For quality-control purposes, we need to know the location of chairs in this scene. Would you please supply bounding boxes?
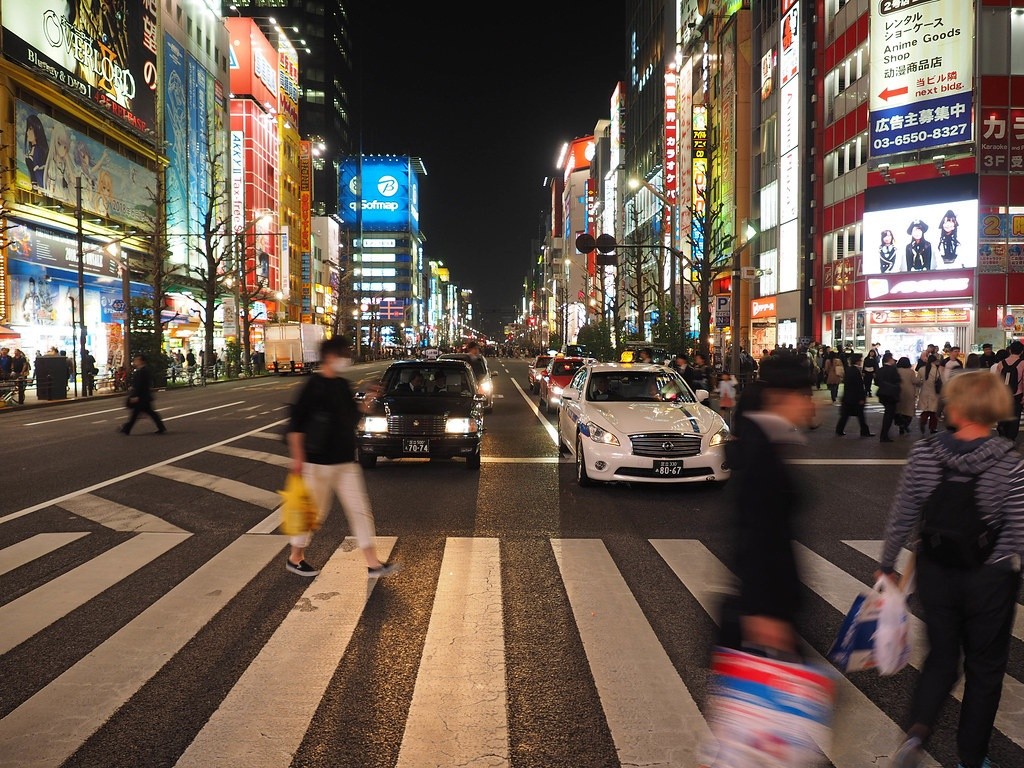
[395,372,411,390]
[446,374,461,385]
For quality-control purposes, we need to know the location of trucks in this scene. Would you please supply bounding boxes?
[263,322,324,376]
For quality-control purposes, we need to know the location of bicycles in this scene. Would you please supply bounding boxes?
[166,367,207,388]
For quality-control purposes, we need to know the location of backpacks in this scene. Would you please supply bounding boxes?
[1001,358,1023,394]
[910,447,1020,622]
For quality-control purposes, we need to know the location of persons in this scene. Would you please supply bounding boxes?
[480,345,528,359]
[118,353,166,436]
[714,371,738,419]
[664,353,715,407]
[86,350,96,396]
[186,349,195,366]
[397,371,426,392]
[284,339,396,578]
[432,371,446,393]
[710,357,813,662]
[388,349,405,360]
[0,348,31,404]
[447,345,461,353]
[416,349,425,358]
[759,340,1024,443]
[463,341,481,379]
[873,370,1024,768]
[33,347,73,390]
[640,348,654,363]
[218,348,226,375]
[162,349,183,369]
[241,348,257,364]
[592,377,613,399]
[877,210,961,273]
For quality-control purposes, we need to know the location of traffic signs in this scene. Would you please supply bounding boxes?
[716,297,730,327]
[868,0,974,162]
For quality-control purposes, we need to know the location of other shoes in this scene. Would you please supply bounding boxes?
[862,432,876,437]
[366,559,400,580]
[115,422,132,436]
[868,392,872,397]
[286,557,318,575]
[155,426,169,433]
[835,430,847,436]
[879,439,894,442]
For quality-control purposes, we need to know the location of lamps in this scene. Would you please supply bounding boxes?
[933,155,950,177]
[878,163,896,184]
[230,5,311,54]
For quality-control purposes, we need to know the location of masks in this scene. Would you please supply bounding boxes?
[331,358,351,373]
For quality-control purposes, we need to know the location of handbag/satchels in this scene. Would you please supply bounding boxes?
[696,646,836,768]
[935,375,943,394]
[835,366,844,377]
[876,578,912,681]
[280,476,321,533]
[829,578,880,675]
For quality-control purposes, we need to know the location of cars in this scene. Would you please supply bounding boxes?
[558,361,731,492]
[528,355,556,395]
[354,360,487,471]
[540,357,597,415]
[430,353,499,415]
